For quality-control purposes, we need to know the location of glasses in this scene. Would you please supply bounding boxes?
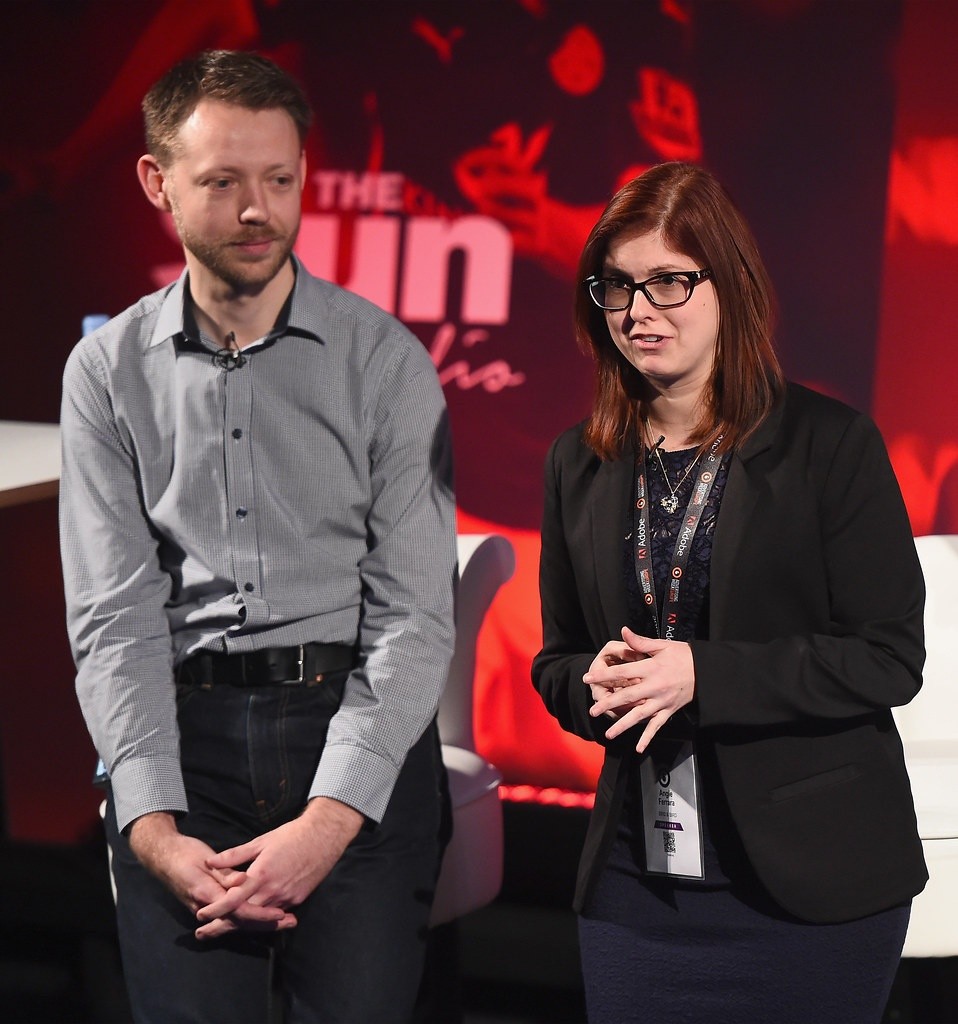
[581,268,714,310]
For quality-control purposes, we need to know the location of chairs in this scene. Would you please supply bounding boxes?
[270,530,520,1024]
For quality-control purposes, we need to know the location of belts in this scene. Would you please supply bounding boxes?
[174,644,359,686]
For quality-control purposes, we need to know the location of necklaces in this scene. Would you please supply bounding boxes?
[646,412,702,512]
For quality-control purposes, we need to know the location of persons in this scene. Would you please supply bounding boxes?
[60,45,461,1024]
[534,164,930,1024]
